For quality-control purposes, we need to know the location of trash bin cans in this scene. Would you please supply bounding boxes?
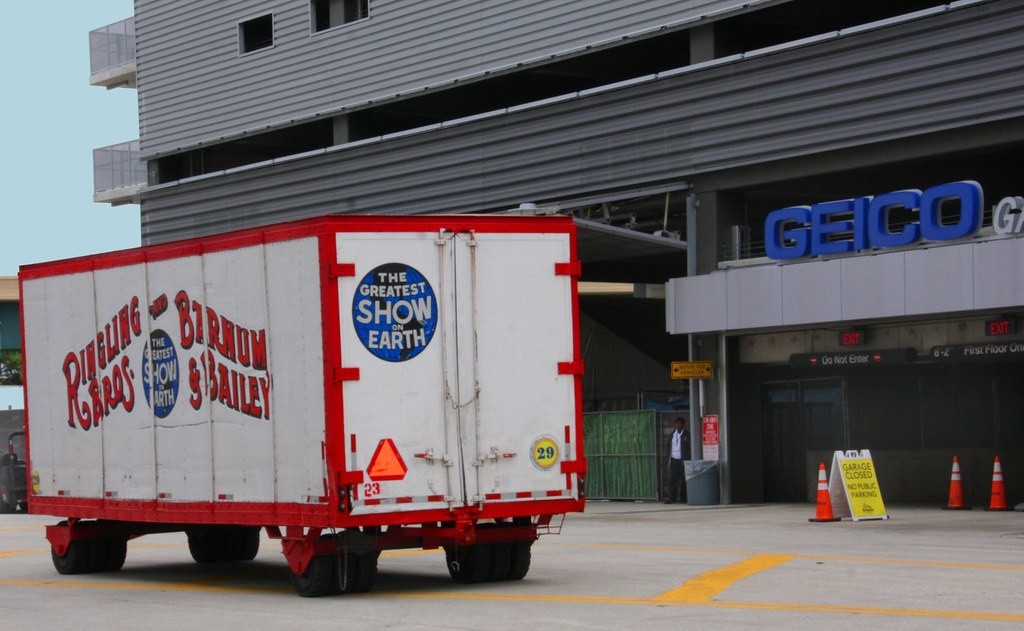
[680,459,720,506]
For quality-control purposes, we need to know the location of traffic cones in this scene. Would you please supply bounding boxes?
[942,456,970,509]
[983,456,1012,511]
[809,462,841,522]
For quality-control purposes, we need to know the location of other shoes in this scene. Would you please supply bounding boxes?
[664,498,673,504]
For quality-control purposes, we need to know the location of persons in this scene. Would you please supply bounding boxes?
[663,417,690,503]
